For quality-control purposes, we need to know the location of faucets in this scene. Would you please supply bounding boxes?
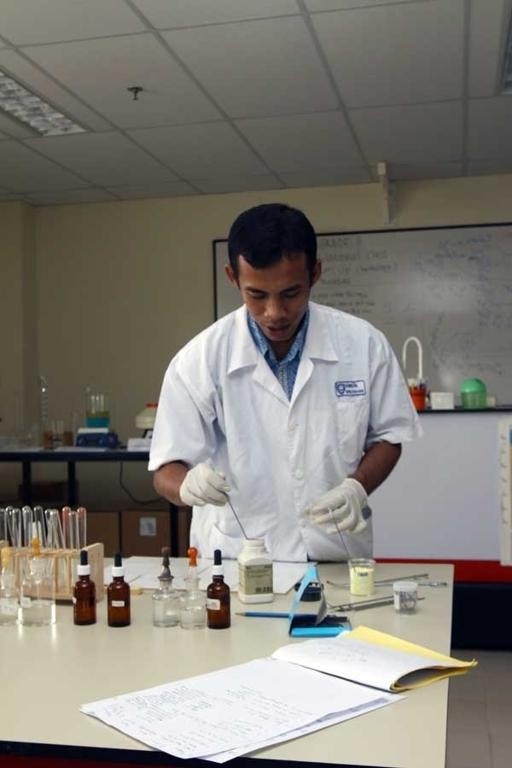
[401,336,423,379]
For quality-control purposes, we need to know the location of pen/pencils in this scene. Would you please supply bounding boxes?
[235,610,291,618]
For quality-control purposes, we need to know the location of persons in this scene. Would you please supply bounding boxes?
[147,200,428,564]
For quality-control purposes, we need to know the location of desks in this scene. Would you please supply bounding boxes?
[1,442,185,557]
[0,554,455,768]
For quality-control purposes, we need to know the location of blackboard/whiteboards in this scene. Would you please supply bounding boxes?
[212,222,510,414]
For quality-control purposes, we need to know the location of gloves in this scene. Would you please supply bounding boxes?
[179,463,231,507]
[310,478,372,535]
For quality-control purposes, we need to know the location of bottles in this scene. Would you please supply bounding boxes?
[2,505,132,628]
[153,544,232,631]
[239,537,273,603]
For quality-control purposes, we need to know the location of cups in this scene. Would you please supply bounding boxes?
[393,580,419,616]
[347,560,375,595]
[406,378,429,411]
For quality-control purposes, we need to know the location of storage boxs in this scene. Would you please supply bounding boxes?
[86,501,191,557]
